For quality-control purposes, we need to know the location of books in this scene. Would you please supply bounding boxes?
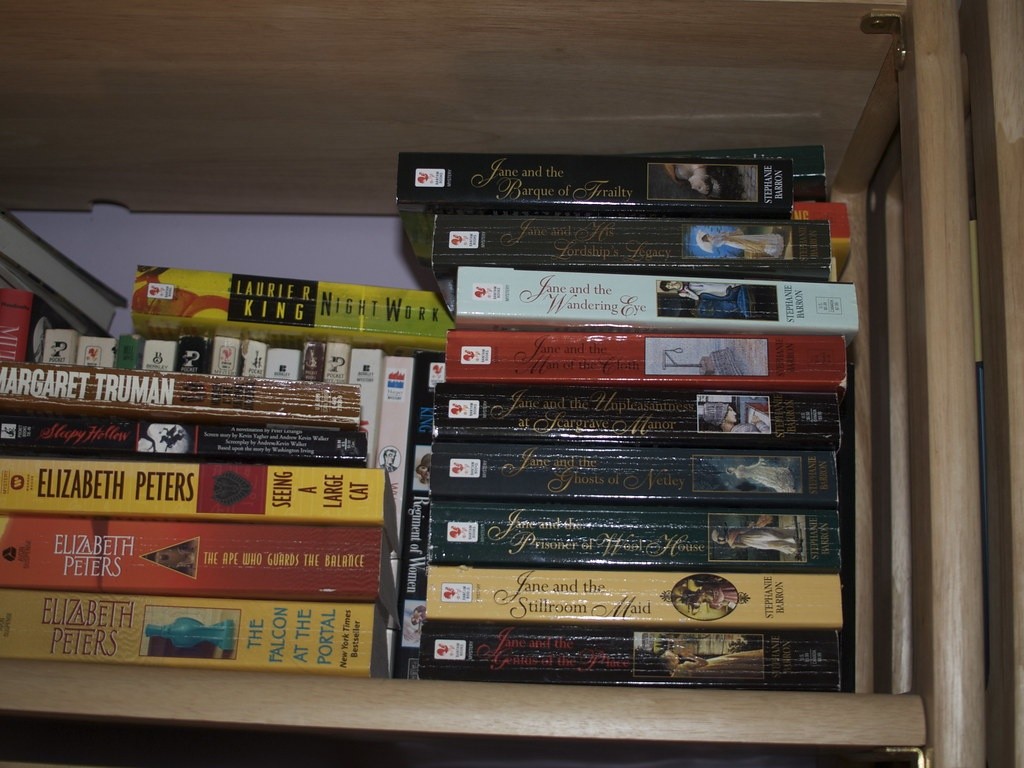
[1,265,455,676]
[395,146,858,692]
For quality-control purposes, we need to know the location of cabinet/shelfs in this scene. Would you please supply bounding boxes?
[0,0,1024,768]
